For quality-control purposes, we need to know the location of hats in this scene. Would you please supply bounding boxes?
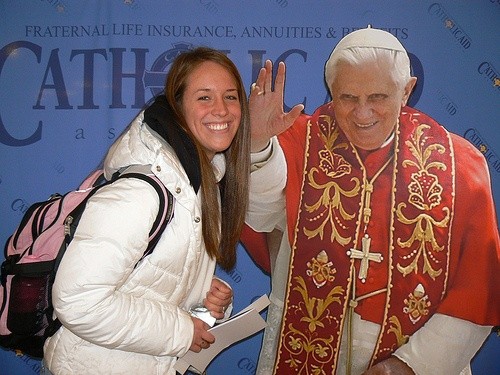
[334,25,407,52]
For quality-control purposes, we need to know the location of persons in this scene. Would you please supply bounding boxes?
[43,46,252,375]
[243,24,500,375]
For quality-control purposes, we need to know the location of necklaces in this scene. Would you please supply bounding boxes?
[346,139,395,280]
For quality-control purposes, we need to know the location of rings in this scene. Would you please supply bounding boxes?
[251,82,265,96]
[222,306,225,313]
[200,340,206,347]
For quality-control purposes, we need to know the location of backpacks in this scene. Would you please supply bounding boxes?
[0,169,176,360]
